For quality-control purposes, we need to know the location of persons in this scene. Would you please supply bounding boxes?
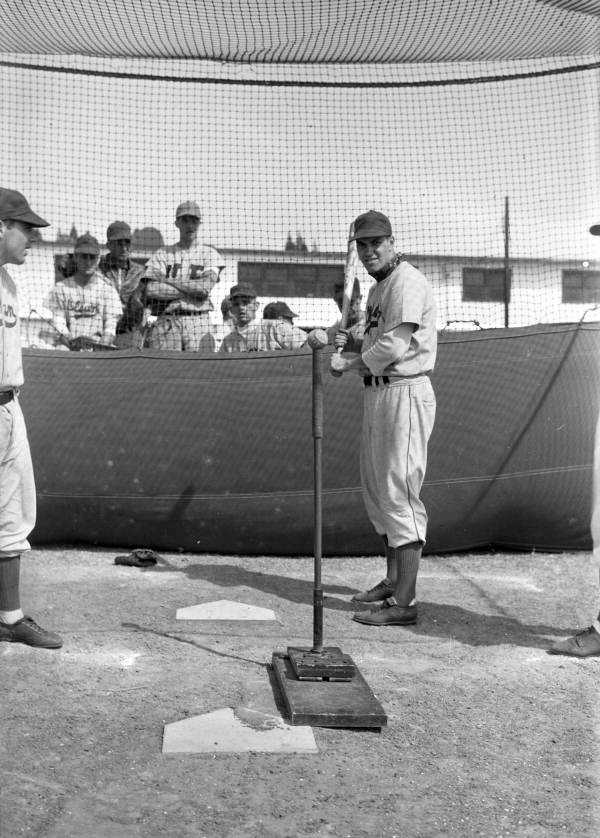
[326,275,367,345]
[329,211,437,625]
[140,200,226,354]
[49,235,123,351]
[263,301,299,325]
[96,221,148,351]
[551,223,599,659]
[219,295,239,339]
[0,186,64,649]
[219,282,310,353]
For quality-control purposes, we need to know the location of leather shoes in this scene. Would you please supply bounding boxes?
[551,625,600,655]
[355,578,395,603]
[354,596,418,626]
[0,616,63,649]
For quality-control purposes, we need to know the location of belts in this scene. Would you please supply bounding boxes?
[164,310,209,316]
[364,376,390,388]
[0,387,20,406]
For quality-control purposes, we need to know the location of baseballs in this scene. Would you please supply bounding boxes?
[308,328,329,349]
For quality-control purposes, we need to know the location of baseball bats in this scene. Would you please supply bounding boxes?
[329,220,360,378]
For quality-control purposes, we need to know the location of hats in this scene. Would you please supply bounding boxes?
[0,186,50,229]
[333,276,361,301]
[107,220,133,243]
[350,209,393,241]
[263,301,299,320]
[230,283,256,301]
[221,295,233,313]
[73,234,101,256]
[176,200,201,220]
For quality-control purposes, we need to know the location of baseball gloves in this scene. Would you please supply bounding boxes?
[114,547,157,567]
